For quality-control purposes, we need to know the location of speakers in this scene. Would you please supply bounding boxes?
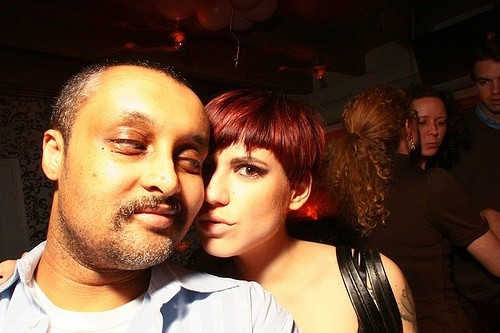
[412,6,500,91]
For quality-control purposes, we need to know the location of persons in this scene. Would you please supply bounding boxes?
[1,91,418,333]
[435,48,500,240]
[327,78,500,333]
[0,59,314,333]
